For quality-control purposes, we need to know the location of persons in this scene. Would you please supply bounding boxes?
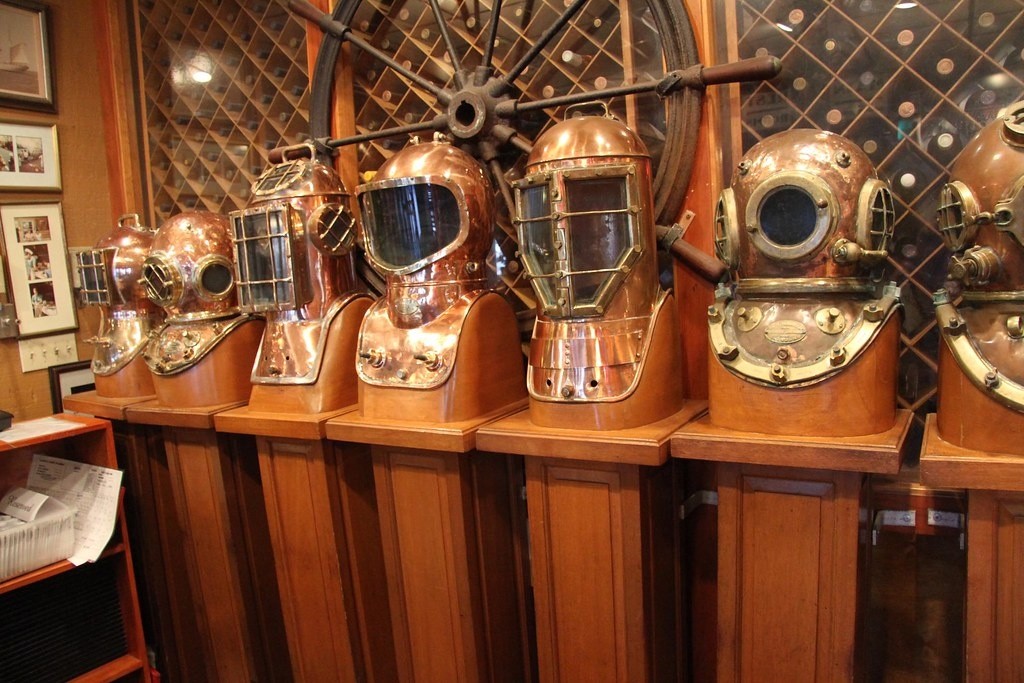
[24,247,39,280]
[25,230,41,241]
[20,150,30,163]
[32,287,46,318]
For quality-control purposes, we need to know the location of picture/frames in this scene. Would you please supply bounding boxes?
[0,1,59,115]
[49,360,96,414]
[0,199,79,340]
[0,117,63,194]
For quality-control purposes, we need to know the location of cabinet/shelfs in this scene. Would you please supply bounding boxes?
[0,411,151,683]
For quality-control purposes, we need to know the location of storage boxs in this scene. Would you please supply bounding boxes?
[0,506,76,583]
